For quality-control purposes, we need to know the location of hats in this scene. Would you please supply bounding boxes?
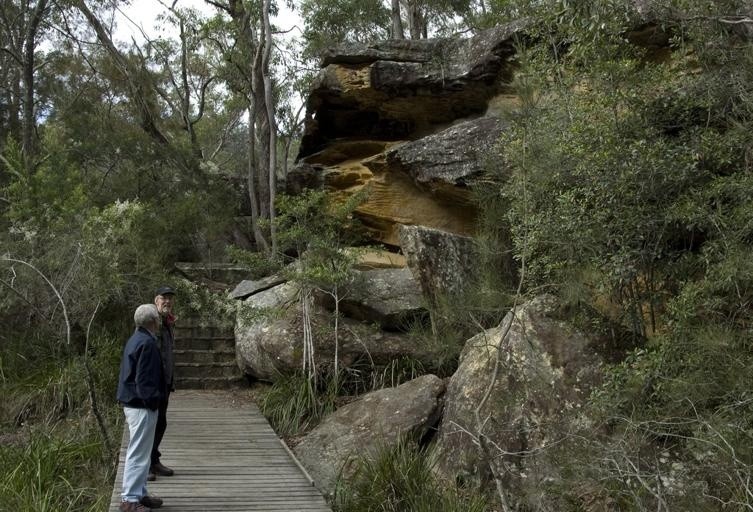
[156,286,175,295]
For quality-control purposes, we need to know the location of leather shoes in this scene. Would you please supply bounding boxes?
[120,496,163,512]
[146,462,175,480]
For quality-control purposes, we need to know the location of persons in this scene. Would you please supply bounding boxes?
[115,286,177,512]
